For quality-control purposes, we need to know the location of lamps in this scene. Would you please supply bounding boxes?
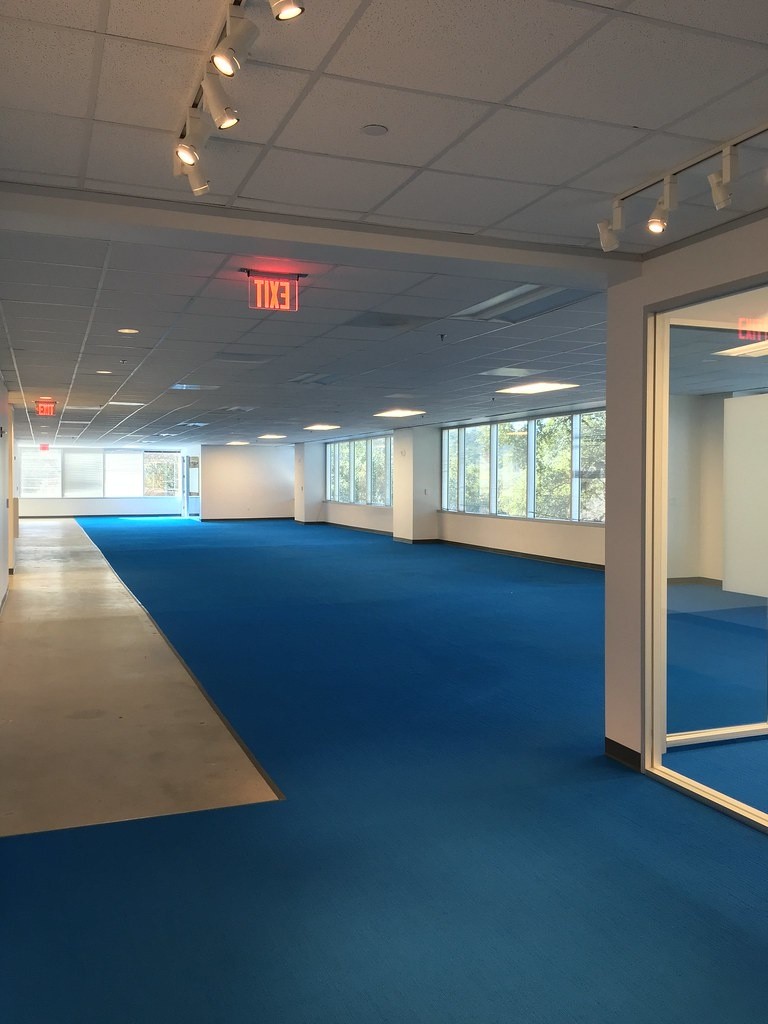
[705,147,741,210]
[210,7,259,78]
[267,0,306,21]
[597,201,626,251]
[197,64,239,130]
[174,109,209,163]
[645,177,679,233]
[179,166,210,196]
